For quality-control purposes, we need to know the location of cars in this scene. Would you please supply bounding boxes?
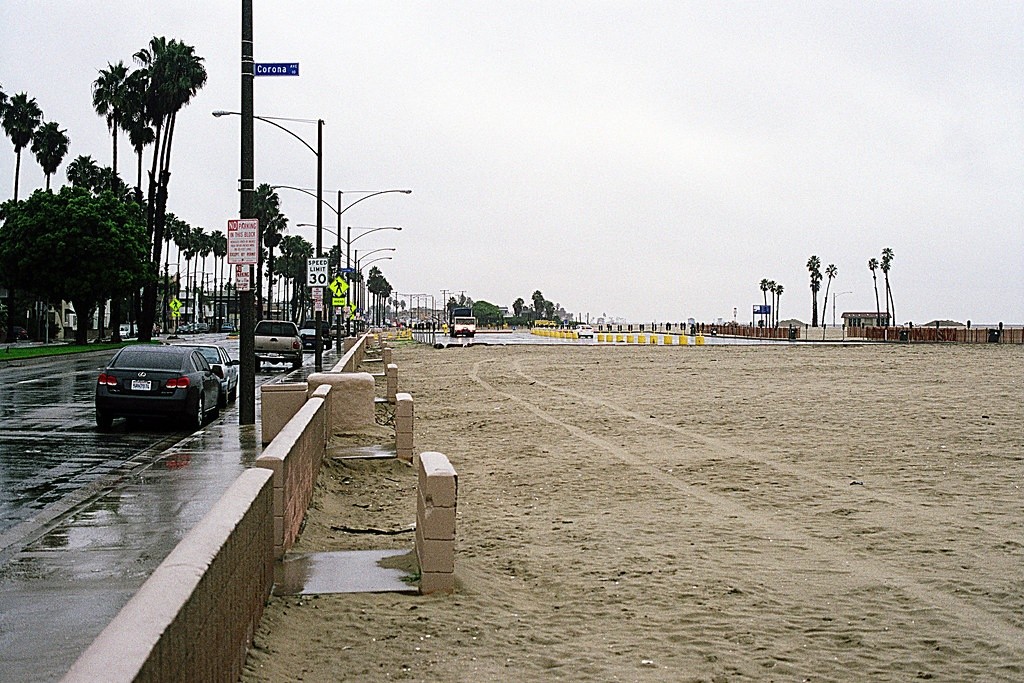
[14,327,28,340]
[576,325,594,339]
[330,320,362,340]
[221,322,234,332]
[170,344,240,408]
[95,344,225,430]
[111,323,210,339]
[298,320,332,350]
[254,320,303,372]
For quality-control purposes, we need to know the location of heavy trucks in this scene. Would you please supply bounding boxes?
[535,320,557,328]
[450,308,478,337]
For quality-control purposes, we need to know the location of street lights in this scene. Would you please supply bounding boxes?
[268,184,412,356]
[212,110,326,369]
[296,223,403,335]
[315,246,397,336]
[327,256,393,331]
